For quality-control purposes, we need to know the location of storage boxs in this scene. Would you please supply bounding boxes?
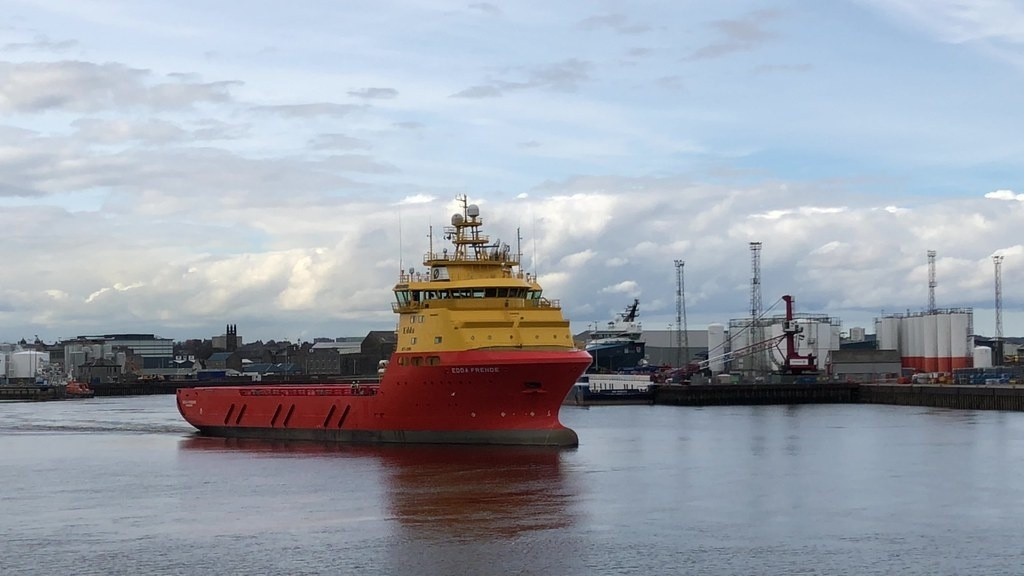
[896,365,1024,386]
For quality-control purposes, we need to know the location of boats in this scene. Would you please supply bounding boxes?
[572,299,652,404]
[177,194,592,447]
